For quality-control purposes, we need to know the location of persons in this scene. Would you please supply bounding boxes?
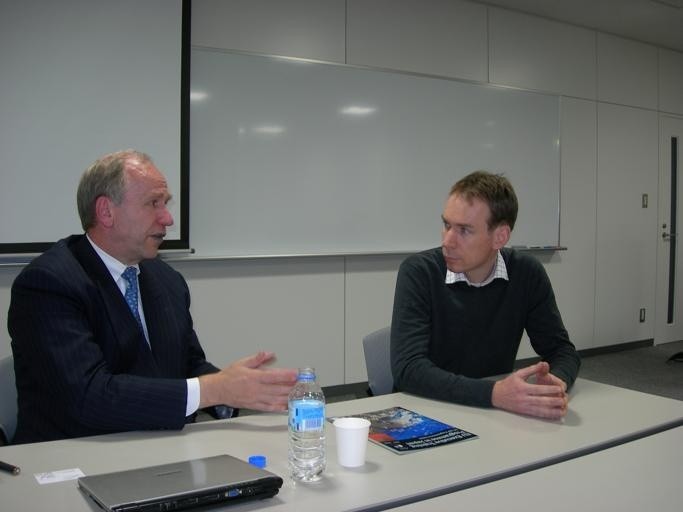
[8,150,299,445]
[391,171,582,420]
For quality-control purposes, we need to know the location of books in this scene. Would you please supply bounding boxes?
[326,406,479,456]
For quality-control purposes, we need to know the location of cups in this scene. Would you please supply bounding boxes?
[332,417,370,466]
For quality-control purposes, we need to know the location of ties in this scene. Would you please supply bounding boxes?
[121,267,142,331]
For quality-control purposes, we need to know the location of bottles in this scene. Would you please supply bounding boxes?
[289,368,327,483]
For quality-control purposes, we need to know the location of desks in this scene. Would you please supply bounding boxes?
[2,370,681,510]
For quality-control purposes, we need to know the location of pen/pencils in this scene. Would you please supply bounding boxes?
[529,247,541,248]
[544,246,557,248]
[511,246,527,248]
[0,461,20,476]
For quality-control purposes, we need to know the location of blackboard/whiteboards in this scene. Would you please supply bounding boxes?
[1,46,567,266]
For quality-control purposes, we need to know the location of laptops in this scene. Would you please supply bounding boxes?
[78,454,283,512]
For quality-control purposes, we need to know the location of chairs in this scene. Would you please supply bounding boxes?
[362,326,395,396]
